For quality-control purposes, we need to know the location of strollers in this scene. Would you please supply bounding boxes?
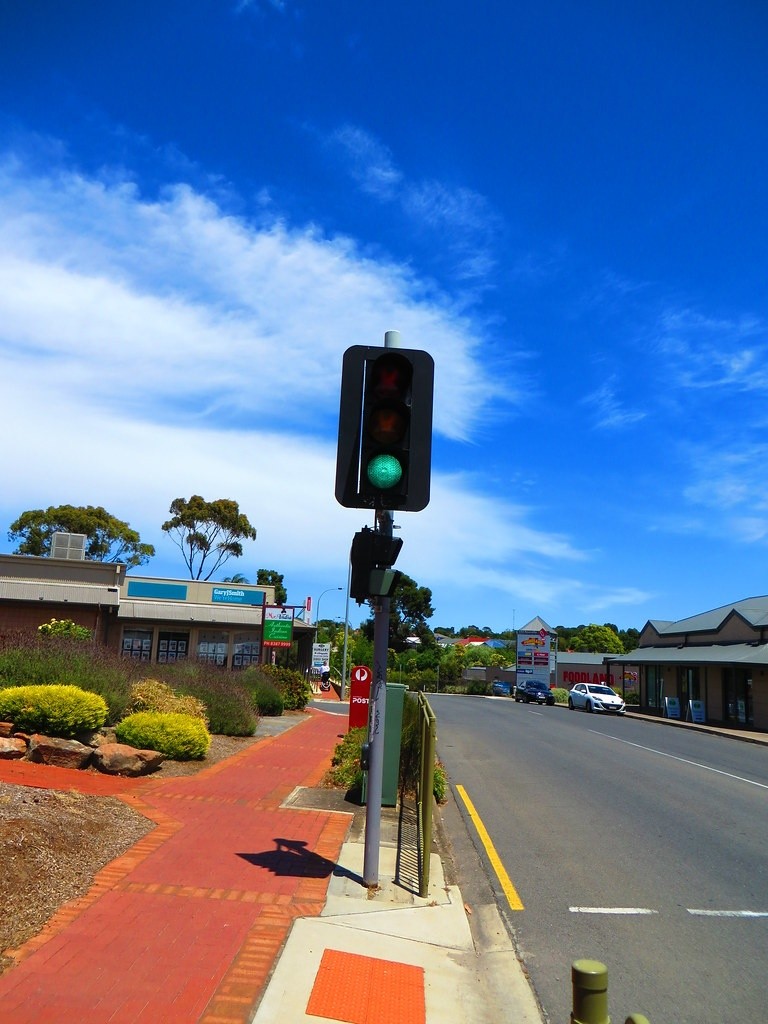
[319,671,331,691]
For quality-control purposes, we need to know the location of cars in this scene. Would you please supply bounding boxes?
[515,679,555,706]
[493,682,509,695]
[568,683,626,715]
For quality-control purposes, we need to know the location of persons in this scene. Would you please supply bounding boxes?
[320,661,331,677]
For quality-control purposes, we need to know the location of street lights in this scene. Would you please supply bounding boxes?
[315,588,343,644]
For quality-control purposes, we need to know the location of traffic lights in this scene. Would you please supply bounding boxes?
[334,345,434,513]
[350,524,404,607]
